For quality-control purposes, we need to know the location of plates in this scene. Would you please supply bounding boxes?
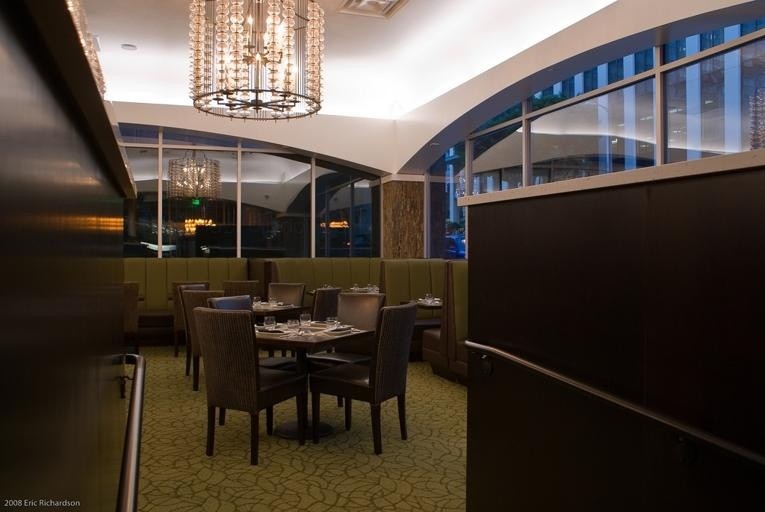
[256,331,290,336]
[325,330,351,335]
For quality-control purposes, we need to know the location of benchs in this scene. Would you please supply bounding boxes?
[123,256,469,387]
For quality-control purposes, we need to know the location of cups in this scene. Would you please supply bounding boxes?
[326,317,337,330]
[253,297,261,305]
[424,293,433,304]
[287,314,312,335]
[264,317,276,330]
[353,287,359,294]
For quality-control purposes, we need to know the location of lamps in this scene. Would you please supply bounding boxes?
[167,142,222,201]
[187,0,325,122]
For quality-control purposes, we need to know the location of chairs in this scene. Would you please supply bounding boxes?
[171,279,415,470]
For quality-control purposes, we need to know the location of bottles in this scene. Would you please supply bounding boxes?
[269,296,277,303]
[368,282,376,293]
